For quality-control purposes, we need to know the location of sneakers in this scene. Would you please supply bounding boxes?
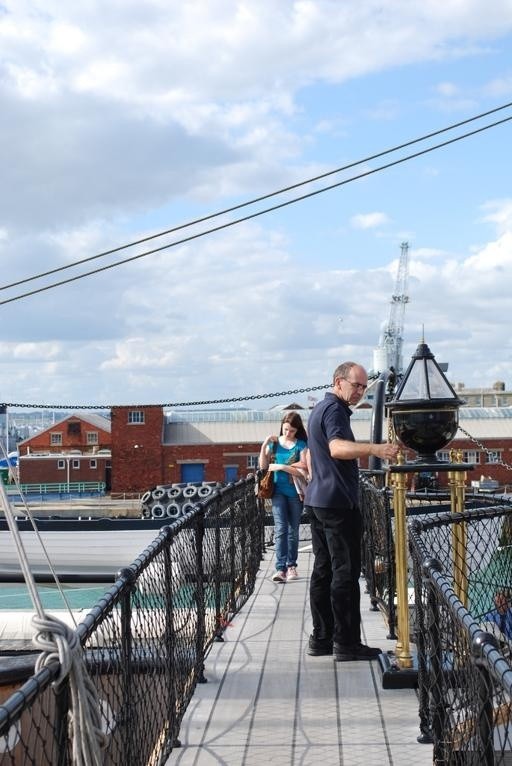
[286,565,298,580]
[332,640,383,662]
[306,633,333,656]
[272,570,287,584]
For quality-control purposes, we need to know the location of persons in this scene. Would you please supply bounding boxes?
[304,361,401,664]
[258,412,309,584]
[479,593,512,643]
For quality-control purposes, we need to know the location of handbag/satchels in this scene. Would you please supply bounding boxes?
[258,435,278,499]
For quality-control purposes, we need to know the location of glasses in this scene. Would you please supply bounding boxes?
[338,377,368,390]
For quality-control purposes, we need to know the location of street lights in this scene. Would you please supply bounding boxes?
[377,321,475,689]
